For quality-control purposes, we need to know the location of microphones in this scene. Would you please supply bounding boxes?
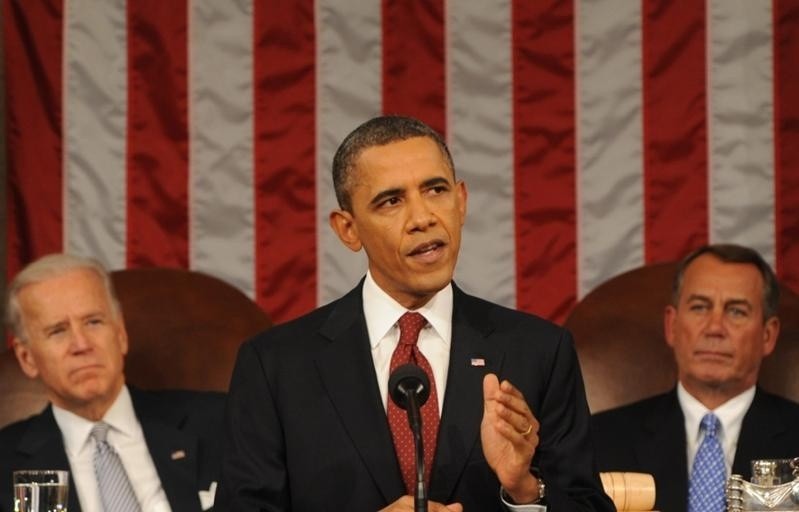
[388,364,431,512]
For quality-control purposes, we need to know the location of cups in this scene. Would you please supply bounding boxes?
[13,470,71,512]
[751,459,799,487]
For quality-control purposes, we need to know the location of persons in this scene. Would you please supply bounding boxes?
[587,244,799,512]
[0,254,230,512]
[223,115,618,512]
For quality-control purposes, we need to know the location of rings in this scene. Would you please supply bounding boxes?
[520,423,533,436]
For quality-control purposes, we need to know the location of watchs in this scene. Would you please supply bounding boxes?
[503,478,546,505]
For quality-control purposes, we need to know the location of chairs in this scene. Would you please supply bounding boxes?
[563,259,798,414]
[0,266,275,429]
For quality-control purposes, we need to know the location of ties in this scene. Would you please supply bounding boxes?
[689,414,727,512]
[386,313,440,497]
[91,423,142,512]
